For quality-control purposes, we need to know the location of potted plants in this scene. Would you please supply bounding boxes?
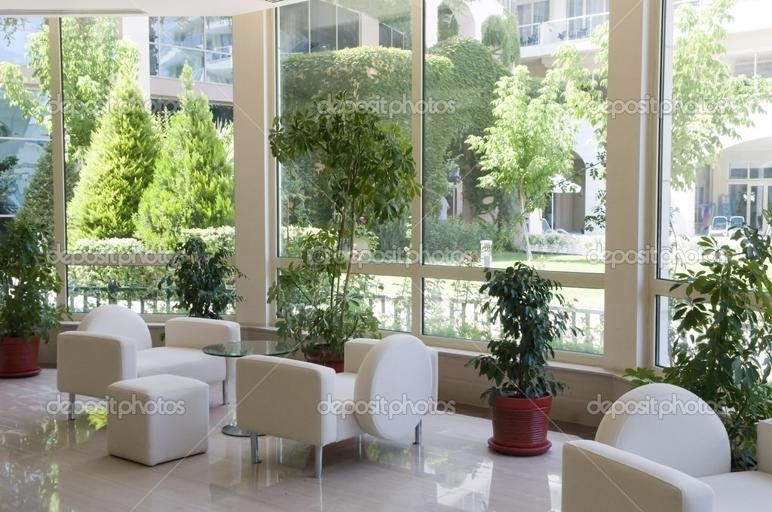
[265,87,424,373]
[464,262,584,454]
[0,210,72,378]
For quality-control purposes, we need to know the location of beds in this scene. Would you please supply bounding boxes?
[203,339,297,437]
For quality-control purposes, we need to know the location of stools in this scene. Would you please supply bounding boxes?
[105,374,210,467]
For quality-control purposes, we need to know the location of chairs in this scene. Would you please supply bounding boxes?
[56,305,240,420]
[561,383,772,512]
[235,335,439,477]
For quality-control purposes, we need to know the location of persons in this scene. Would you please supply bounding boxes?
[437,193,452,221]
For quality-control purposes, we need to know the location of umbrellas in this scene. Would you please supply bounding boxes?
[533,171,582,236]
